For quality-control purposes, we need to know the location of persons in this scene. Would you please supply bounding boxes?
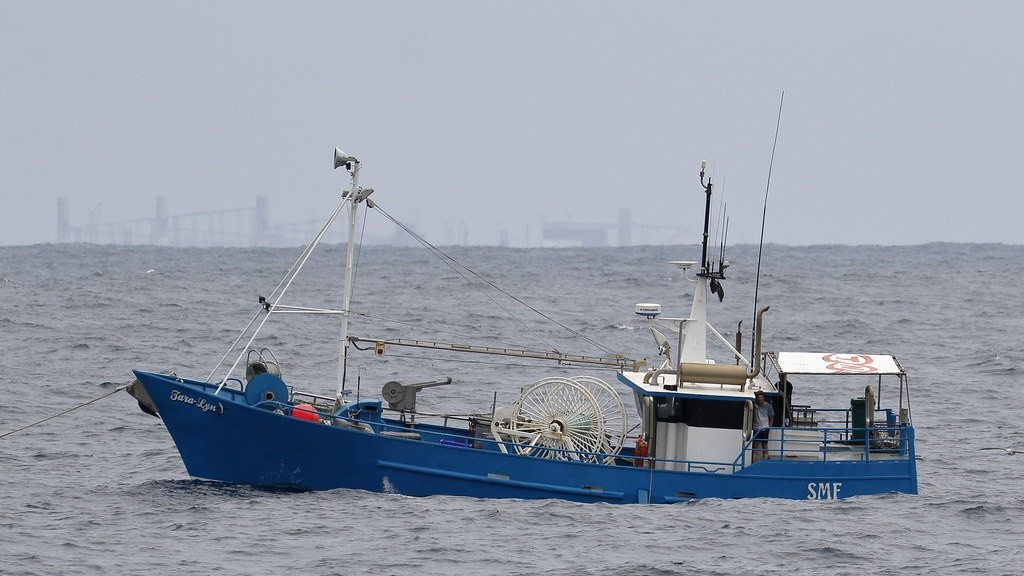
[750,391,775,465]
[772,371,794,427]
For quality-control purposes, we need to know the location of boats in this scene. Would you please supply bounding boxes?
[132,91,920,508]
